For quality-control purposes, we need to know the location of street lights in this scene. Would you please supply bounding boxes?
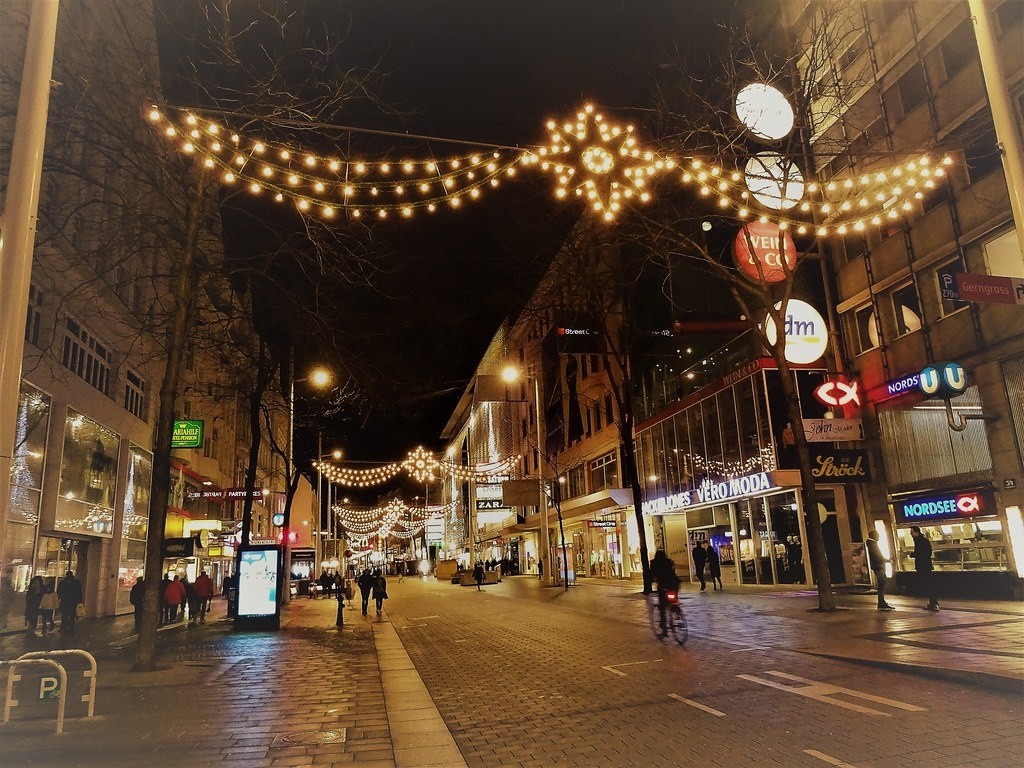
[331,486,349,539]
[415,484,431,575]
[501,365,553,586]
[312,432,343,578]
[447,439,475,569]
[284,366,334,605]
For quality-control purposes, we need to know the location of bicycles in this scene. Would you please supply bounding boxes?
[640,588,689,646]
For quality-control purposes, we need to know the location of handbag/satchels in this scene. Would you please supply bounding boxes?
[76,604,86,620]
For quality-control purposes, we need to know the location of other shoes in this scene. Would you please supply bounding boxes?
[878,605,895,610]
[41,630,46,634]
[700,587,705,590]
[923,604,939,610]
[714,586,716,590]
[49,624,55,631]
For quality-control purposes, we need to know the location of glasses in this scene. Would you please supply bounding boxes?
[911,532,915,533]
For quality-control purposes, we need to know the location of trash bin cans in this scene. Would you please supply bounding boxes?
[227,588,235,618]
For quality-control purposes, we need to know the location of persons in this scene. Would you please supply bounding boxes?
[908,526,941,612]
[485,556,509,576]
[702,539,724,592]
[358,569,388,616]
[320,571,342,596]
[130,577,143,629]
[457,563,463,571]
[160,569,213,626]
[398,572,404,583]
[538,557,543,580]
[865,530,896,611]
[223,570,236,600]
[782,422,796,448]
[23,570,82,634]
[472,559,486,591]
[644,550,683,637]
[692,540,707,591]
